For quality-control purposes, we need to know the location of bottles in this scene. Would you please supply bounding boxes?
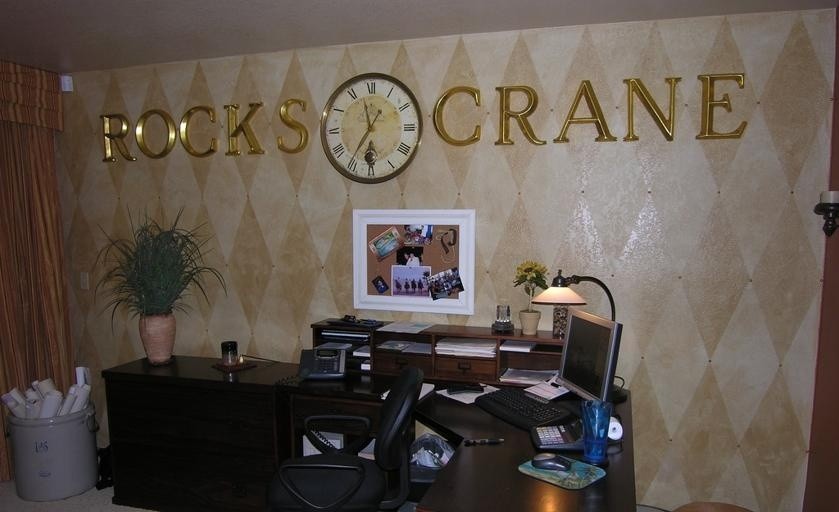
[224,372,238,382]
[239,354,244,364]
[221,340,240,367]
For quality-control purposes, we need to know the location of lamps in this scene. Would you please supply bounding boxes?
[812,190,839,237]
[530,267,628,404]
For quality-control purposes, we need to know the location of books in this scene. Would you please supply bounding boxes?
[352,346,370,356]
[499,340,536,352]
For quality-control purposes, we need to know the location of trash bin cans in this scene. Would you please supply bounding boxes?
[408,433,456,503]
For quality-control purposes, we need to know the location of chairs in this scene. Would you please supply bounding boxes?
[268,364,425,511]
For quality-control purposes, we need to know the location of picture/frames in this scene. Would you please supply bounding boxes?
[353,209,475,316]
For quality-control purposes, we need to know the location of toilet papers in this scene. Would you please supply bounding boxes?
[607,416,623,443]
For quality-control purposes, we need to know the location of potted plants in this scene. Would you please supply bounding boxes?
[87,194,230,368]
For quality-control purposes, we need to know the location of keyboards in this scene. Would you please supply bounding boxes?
[475,388,571,432]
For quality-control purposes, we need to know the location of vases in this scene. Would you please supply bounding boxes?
[518,309,542,336]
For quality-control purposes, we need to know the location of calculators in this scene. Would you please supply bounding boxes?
[531,424,584,449]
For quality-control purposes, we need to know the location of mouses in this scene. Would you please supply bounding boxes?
[532,453,571,472]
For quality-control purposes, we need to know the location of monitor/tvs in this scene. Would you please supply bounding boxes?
[557,308,623,423]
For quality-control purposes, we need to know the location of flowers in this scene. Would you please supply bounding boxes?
[511,260,549,312]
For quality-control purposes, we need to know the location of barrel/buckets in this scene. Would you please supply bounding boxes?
[4,404,100,501]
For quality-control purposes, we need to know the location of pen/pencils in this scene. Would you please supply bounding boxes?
[464,438,505,447]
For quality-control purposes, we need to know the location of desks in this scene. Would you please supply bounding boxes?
[100,352,301,512]
[286,316,637,512]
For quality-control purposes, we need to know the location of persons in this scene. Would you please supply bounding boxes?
[403,253,410,265]
[404,276,410,293]
[418,279,423,295]
[395,277,402,294]
[411,278,417,292]
[406,253,419,266]
[374,278,387,293]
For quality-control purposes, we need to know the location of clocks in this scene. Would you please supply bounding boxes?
[319,72,424,185]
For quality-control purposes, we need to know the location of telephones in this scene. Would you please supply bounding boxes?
[297,348,346,380]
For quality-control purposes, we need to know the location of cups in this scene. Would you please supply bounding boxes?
[579,399,618,465]
